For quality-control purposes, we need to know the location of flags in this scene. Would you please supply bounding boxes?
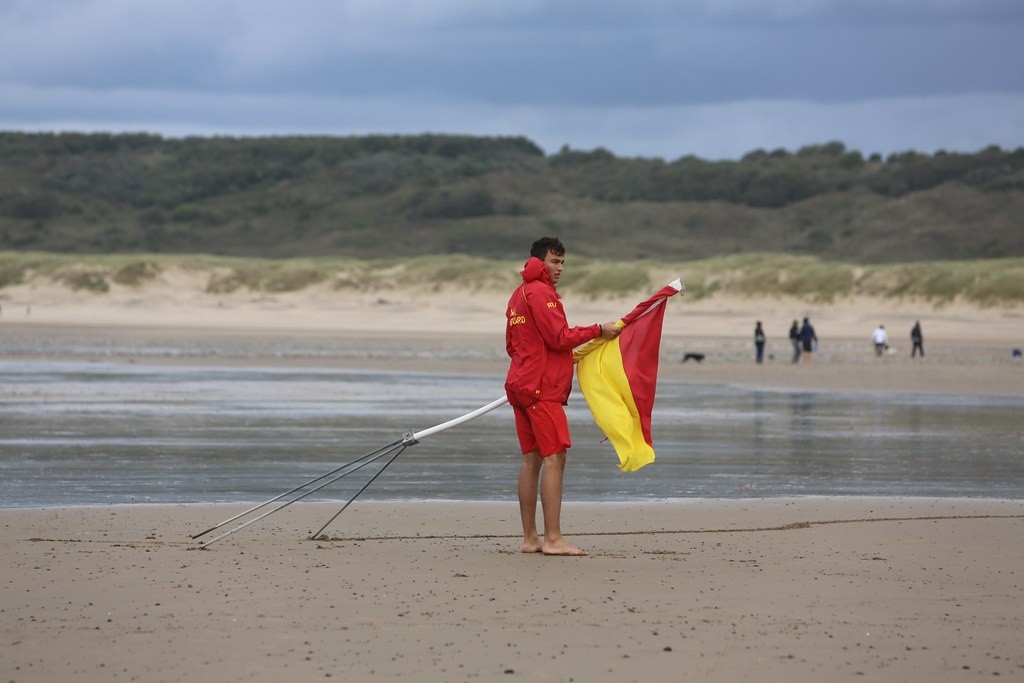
[572,278,682,472]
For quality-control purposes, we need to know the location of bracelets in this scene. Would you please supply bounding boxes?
[599,324,603,337]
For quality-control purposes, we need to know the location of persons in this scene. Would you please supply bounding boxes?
[911,322,924,358]
[504,236,622,555]
[755,322,766,362]
[789,316,818,364]
[872,324,889,357]
